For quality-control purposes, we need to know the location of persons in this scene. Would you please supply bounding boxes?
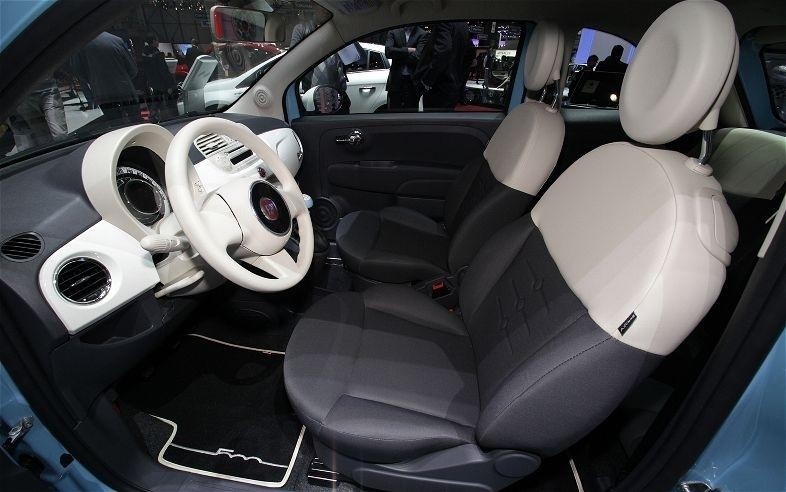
[0,31,203,156]
[569,45,626,98]
[289,9,349,113]
[385,22,496,112]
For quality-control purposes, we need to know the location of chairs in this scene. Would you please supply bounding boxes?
[333,23,567,282]
[705,128,784,272]
[280,2,741,492]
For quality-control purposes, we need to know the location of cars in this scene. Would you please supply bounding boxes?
[174,41,424,116]
[171,36,284,93]
